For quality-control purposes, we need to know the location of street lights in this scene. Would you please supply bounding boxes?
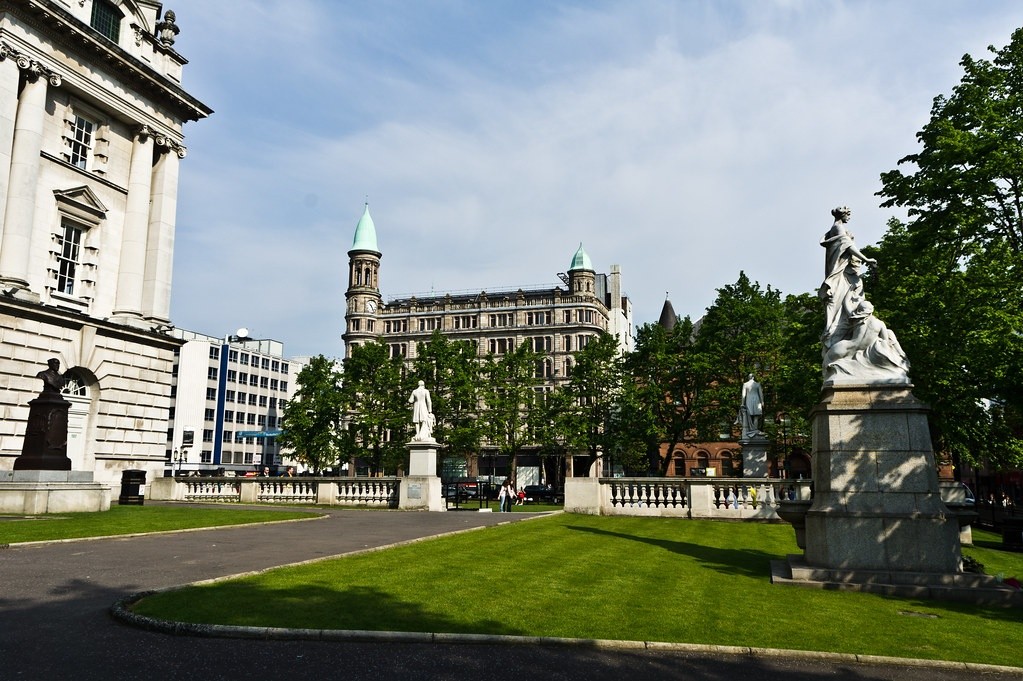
[210,327,252,464]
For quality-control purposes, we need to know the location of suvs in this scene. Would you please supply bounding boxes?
[517,484,555,506]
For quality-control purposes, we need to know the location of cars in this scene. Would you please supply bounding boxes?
[441,483,503,504]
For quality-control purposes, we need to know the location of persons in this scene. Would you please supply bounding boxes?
[498,479,518,513]
[283,466,294,477]
[193,471,200,477]
[259,467,273,477]
[409,380,436,435]
[515,487,526,506]
[36,358,65,393]
[741,374,764,430]
[818,207,910,376]
